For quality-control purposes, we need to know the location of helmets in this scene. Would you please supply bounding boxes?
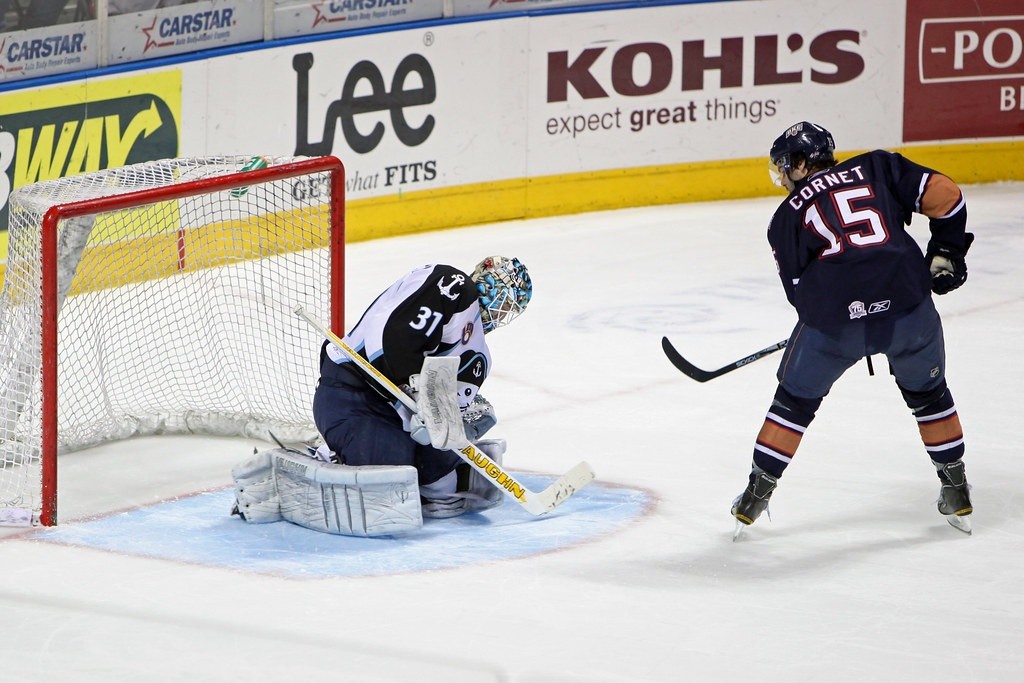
[469,255,532,333]
[770,122,837,170]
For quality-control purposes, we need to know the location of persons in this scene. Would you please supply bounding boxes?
[228,255,531,538]
[729,121,975,538]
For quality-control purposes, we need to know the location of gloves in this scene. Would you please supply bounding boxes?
[924,245,969,294]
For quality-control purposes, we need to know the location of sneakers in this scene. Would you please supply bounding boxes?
[731,472,779,542]
[937,463,974,535]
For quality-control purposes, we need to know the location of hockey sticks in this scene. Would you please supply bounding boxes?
[660,269,955,384]
[291,302,598,519]
